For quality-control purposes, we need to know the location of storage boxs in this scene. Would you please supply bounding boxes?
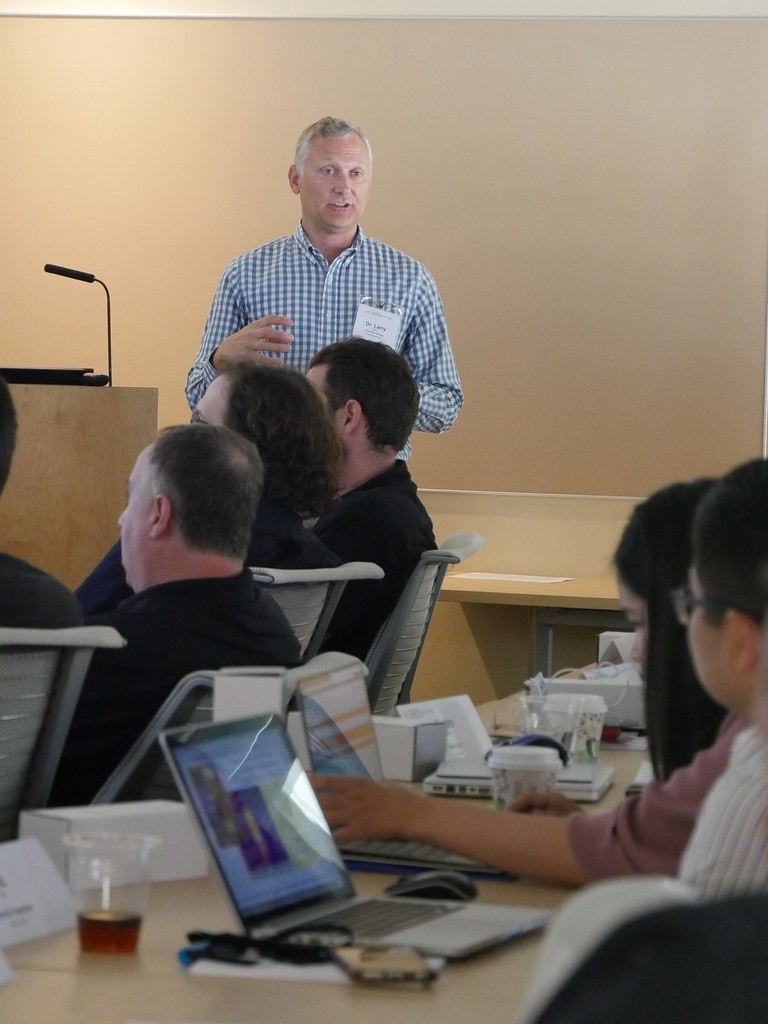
[374,710,454,780]
[16,798,216,890]
[597,631,638,671]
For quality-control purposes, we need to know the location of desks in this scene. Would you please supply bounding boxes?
[0,686,657,1024]
[437,570,635,678]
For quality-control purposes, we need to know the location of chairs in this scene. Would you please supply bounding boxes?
[365,531,488,717]
[0,625,128,842]
[88,651,370,805]
[248,561,385,663]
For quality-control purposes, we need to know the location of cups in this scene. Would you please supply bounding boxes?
[517,695,583,763]
[489,746,562,812]
[64,834,160,953]
[543,694,608,763]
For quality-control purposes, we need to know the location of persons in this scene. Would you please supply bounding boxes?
[184,115,465,461]
[306,458,768,900]
[0,336,438,806]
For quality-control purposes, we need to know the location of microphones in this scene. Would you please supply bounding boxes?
[43,264,112,387]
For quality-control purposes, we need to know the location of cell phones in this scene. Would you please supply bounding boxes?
[357,946,436,983]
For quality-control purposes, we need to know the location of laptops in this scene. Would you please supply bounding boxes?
[159,711,554,958]
[424,760,617,801]
[296,662,520,880]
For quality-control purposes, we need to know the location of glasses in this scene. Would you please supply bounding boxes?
[672,587,714,624]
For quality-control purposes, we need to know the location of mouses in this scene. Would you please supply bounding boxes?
[485,734,569,765]
[385,872,479,902]
